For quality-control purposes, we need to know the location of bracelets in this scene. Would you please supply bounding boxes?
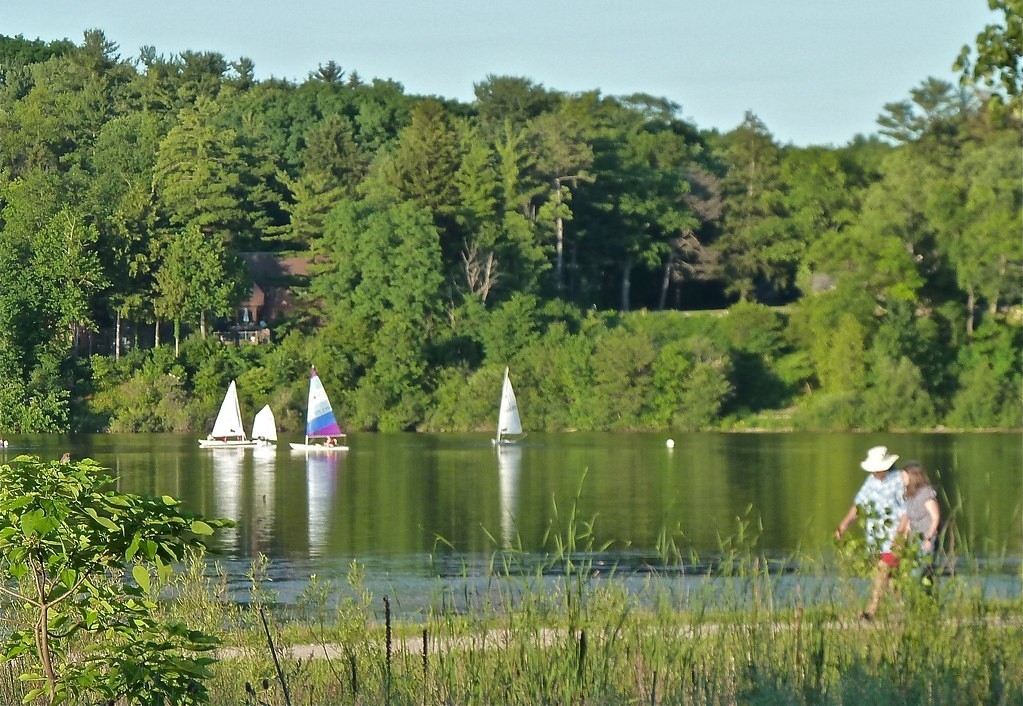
[925,538,930,541]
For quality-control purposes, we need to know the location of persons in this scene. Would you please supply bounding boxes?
[324,436,337,445]
[249,321,254,325]
[221,437,228,441]
[207,432,216,441]
[836,445,901,622]
[891,462,941,597]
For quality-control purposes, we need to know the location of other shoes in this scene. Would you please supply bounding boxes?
[860,611,874,621]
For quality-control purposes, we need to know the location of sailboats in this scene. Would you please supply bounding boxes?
[196,379,256,450]
[249,402,280,452]
[286,364,349,453]
[490,369,530,448]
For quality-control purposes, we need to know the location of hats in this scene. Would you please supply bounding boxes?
[860,446,899,472]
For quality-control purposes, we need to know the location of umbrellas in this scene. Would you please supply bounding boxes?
[243,307,249,322]
